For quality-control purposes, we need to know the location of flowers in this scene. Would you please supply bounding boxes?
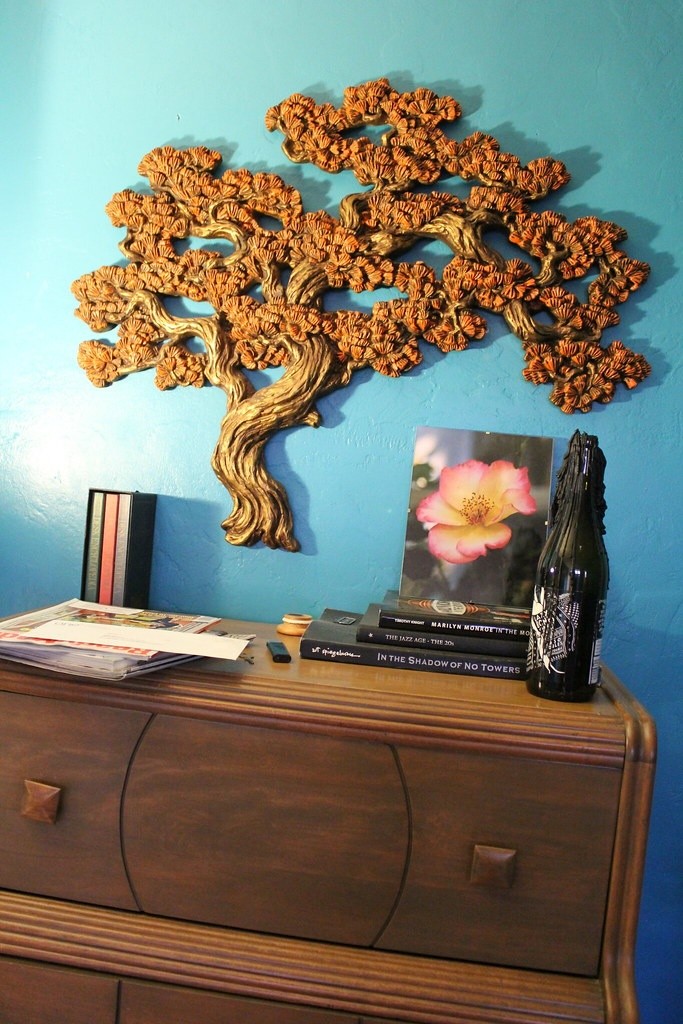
[416,459,538,566]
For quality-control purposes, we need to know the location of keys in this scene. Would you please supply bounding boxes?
[240,652,255,664]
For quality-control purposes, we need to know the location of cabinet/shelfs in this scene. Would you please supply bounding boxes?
[0,607,656,1024]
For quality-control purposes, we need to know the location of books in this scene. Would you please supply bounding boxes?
[0,598,257,681]
[301,589,531,680]
[85,492,130,608]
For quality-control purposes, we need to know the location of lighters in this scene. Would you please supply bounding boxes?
[267,640,291,663]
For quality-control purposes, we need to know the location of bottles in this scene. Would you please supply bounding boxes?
[526,435,609,704]
